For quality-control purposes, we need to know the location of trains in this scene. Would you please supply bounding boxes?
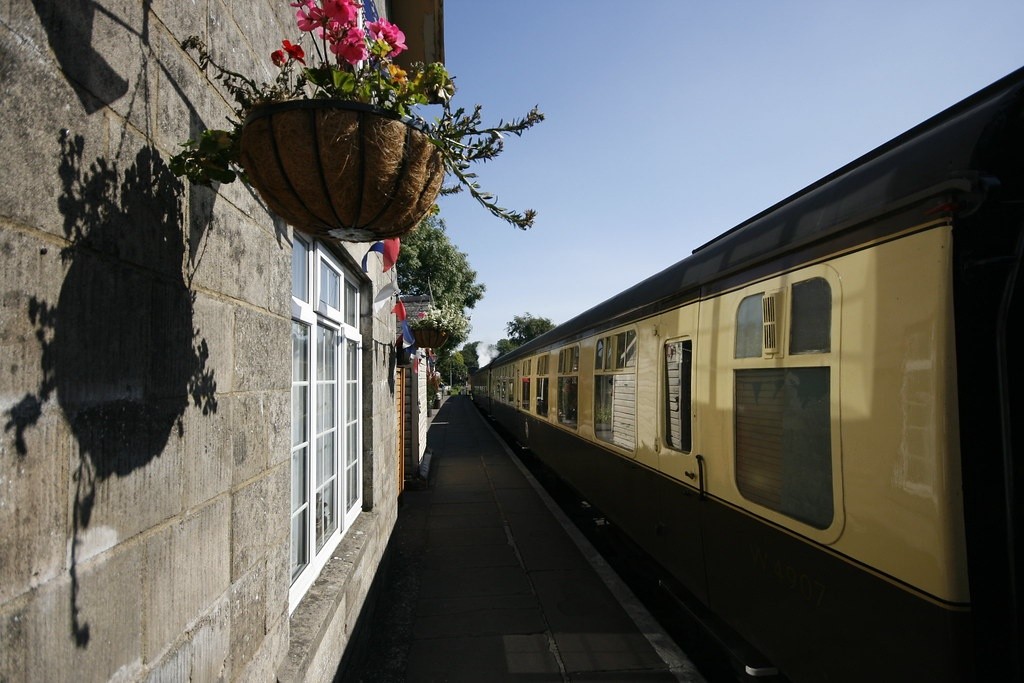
[468,62,1023,683]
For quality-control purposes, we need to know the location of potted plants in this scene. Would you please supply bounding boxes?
[426,369,442,418]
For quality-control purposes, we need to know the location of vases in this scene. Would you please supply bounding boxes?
[412,327,448,348]
[239,98,445,243]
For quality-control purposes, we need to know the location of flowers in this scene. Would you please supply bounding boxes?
[169,0,546,230]
[408,304,459,330]
